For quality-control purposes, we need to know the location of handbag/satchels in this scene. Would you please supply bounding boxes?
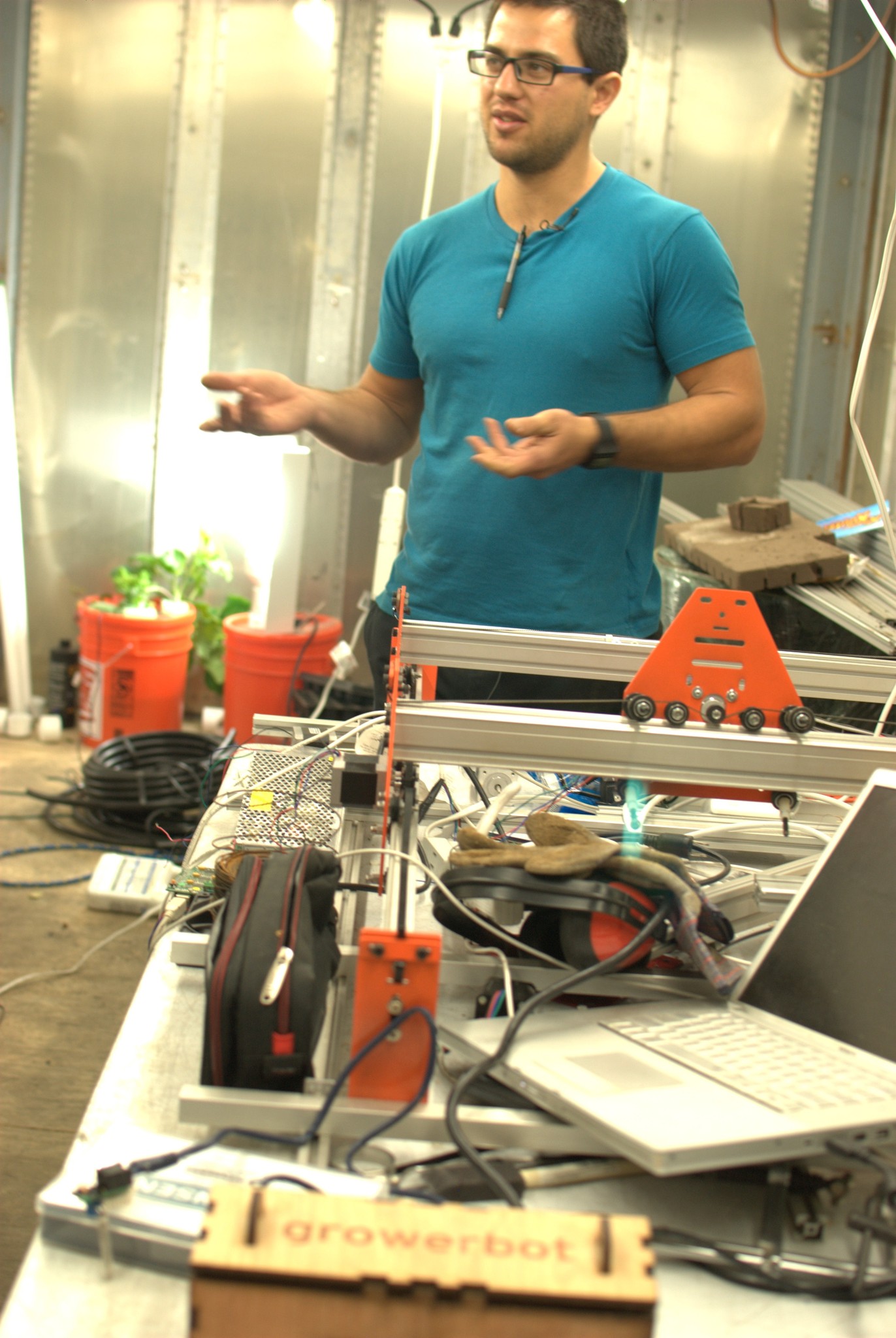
[199,845,342,1092]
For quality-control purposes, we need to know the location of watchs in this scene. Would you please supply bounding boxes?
[577,412,620,470]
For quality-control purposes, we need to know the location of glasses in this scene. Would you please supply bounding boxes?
[467,50,597,85]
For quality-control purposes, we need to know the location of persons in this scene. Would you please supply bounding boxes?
[197,0,766,714]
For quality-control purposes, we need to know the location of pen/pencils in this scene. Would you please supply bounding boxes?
[495,223,528,322]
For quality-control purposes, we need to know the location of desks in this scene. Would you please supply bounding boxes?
[0,733,896,1337]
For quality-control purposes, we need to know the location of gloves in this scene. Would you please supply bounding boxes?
[450,813,746,987]
